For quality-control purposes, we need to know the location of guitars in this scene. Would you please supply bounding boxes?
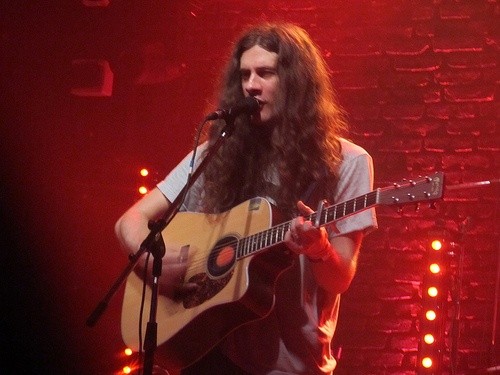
[120,157,447,370]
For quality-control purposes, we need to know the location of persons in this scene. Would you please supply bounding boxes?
[114,23,380,375]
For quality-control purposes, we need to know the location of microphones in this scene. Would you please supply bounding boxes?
[206,96,260,121]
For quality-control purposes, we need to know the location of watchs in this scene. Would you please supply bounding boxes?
[304,248,334,265]
